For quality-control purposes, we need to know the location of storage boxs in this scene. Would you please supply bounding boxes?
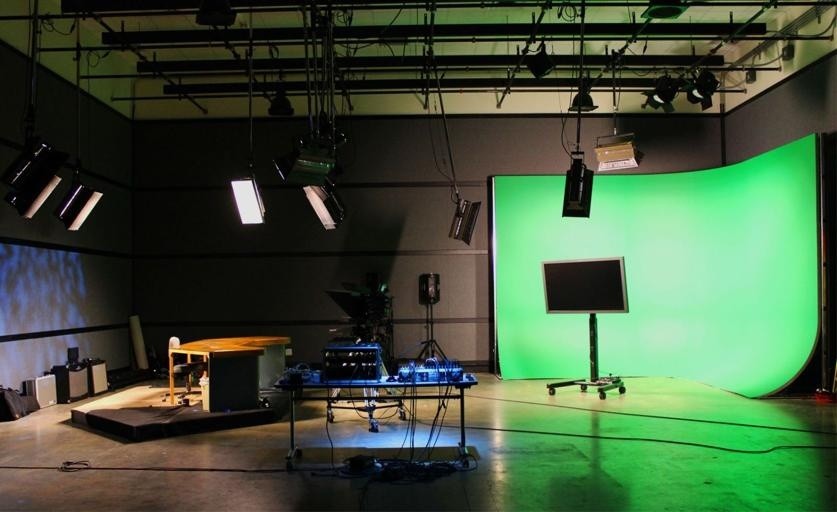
[88,359,111,394]
[54,365,90,404]
[25,375,58,407]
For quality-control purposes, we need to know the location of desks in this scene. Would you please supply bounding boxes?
[282,366,478,470]
[167,333,291,413]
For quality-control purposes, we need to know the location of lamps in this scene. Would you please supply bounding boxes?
[593,38,645,170]
[303,2,348,232]
[5,0,63,221]
[228,1,269,226]
[415,271,451,367]
[561,0,595,218]
[53,1,105,234]
[641,0,777,111]
[424,0,483,245]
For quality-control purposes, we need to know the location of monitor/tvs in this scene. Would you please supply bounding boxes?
[541,256,629,314]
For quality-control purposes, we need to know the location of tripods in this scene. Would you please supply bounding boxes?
[416,304,448,362]
[327,341,406,432]
